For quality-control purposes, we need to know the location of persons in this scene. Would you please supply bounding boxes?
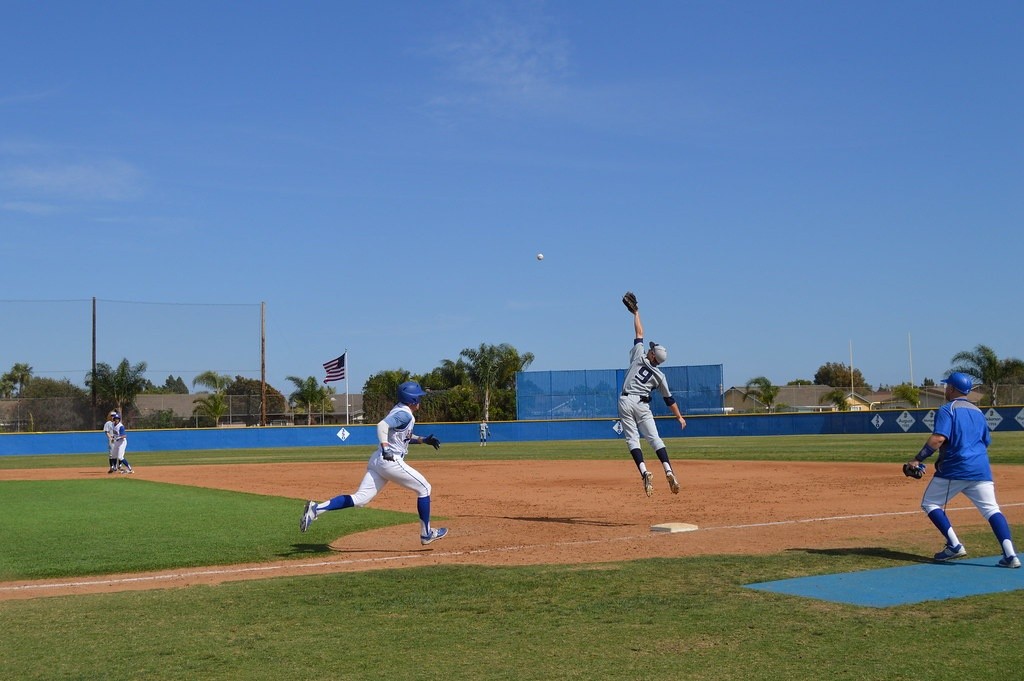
[300,380,449,545]
[618,291,686,497]
[104,412,135,474]
[906,372,1022,567]
[479,419,491,446]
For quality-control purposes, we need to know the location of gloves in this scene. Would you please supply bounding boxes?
[422,434,440,450]
[382,446,395,462]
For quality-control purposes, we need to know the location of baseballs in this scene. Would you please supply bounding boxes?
[537,254,543,260]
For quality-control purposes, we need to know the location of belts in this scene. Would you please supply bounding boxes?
[622,393,642,398]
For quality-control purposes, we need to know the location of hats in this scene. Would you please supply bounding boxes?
[940,371,973,395]
[114,414,121,419]
[111,412,116,416]
[649,341,667,364]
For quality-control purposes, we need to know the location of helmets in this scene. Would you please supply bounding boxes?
[397,382,428,406]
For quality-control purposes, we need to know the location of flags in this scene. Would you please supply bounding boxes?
[323,353,345,384]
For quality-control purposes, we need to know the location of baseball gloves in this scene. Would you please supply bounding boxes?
[902,464,927,479]
[621,291,639,315]
[488,432,491,436]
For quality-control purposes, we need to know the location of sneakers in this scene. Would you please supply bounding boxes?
[299,500,318,533]
[999,554,1021,568]
[420,528,448,545]
[934,544,967,561]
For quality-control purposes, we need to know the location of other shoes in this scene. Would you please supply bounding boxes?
[666,470,680,494]
[642,471,654,497]
